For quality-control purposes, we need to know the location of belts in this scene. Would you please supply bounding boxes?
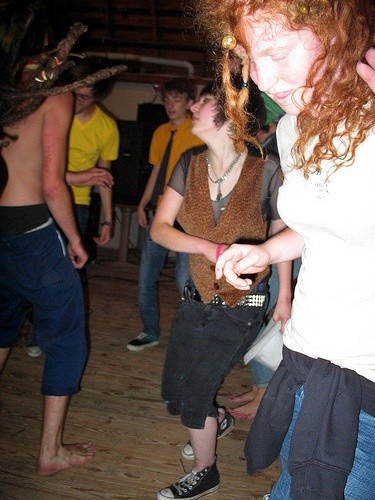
[183,281,270,308]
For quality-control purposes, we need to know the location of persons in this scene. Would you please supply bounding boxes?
[190,0,375,500]
[0,56,119,476]
[127,72,293,499]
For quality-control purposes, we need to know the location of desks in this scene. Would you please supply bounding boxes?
[110,203,150,267]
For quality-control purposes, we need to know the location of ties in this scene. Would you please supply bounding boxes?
[150,129,177,211]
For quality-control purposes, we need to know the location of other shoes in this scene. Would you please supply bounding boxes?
[263,494,270,500]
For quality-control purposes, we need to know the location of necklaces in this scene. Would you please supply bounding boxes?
[205,151,242,200]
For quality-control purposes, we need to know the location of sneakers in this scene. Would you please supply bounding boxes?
[182,408,235,460]
[25,323,43,358]
[127,331,159,351]
[157,456,220,500]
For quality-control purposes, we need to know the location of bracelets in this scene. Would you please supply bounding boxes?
[215,242,223,261]
[101,221,113,227]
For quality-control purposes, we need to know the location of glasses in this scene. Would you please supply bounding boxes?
[74,94,94,100]
